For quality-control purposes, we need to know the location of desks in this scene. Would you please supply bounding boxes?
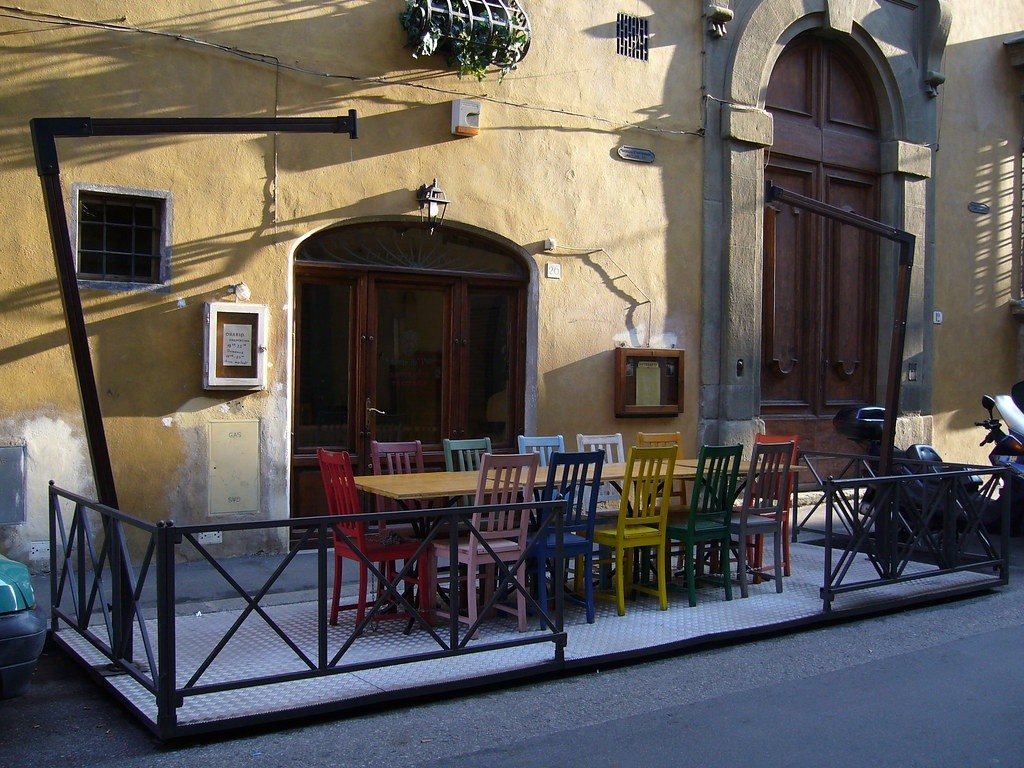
[331,458,810,634]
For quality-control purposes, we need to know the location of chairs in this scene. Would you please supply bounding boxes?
[317,432,798,639]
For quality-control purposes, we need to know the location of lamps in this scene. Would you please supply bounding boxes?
[416,177,451,235]
[227,281,251,300]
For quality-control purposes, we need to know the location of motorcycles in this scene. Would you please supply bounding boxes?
[833,395,1024,552]
